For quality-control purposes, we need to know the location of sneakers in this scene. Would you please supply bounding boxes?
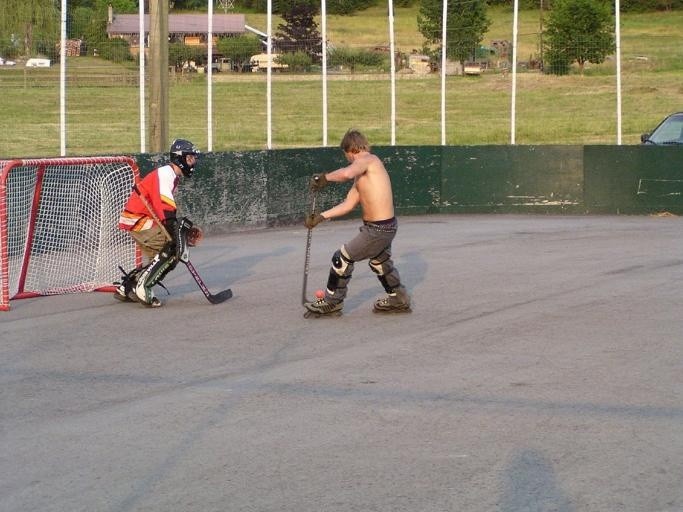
[374,296,409,312]
[304,296,344,314]
[113,284,141,303]
[126,285,162,308]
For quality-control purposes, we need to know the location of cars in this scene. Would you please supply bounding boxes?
[464,62,481,75]
[1,58,15,65]
[641,111,683,145]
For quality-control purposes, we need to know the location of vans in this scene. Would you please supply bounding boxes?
[26,58,51,68]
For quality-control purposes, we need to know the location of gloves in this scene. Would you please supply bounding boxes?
[303,213,324,229]
[308,173,328,192]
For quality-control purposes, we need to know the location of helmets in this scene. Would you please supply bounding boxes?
[169,138,200,179]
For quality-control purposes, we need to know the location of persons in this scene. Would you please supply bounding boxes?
[110,136,205,310]
[298,128,411,314]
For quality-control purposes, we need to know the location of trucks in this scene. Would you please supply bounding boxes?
[205,57,258,74]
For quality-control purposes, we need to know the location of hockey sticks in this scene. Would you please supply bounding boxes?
[131,184,232,303]
[303,177,320,305]
